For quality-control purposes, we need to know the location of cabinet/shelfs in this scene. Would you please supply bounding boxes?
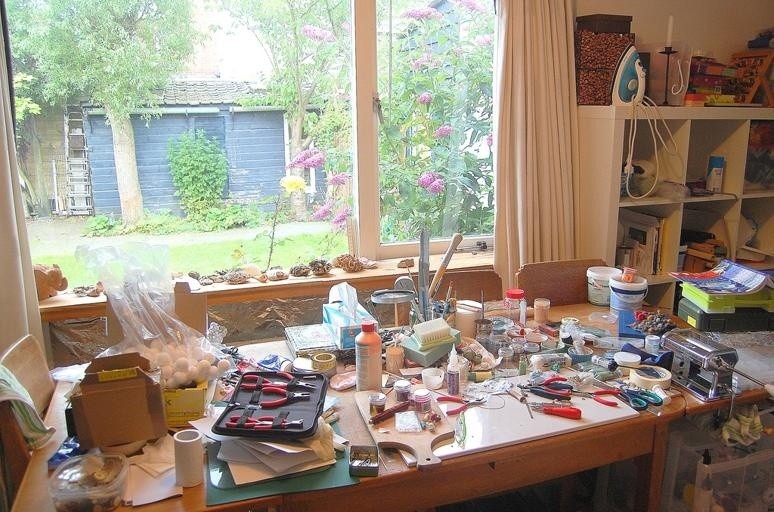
[354,321,383,390]
[502,287,527,328]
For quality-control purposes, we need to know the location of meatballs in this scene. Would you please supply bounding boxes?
[526,402,580,419]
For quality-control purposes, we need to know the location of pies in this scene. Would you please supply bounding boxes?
[480,288,484,324]
[429,233,462,320]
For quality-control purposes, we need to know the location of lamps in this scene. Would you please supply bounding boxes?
[515,259,607,307]
[0,335,56,512]
[408,270,502,307]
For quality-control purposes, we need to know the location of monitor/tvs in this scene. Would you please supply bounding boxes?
[615,209,774,298]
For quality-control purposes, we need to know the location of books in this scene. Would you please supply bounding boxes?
[70,353,169,451]
[576,14,633,34]
[576,70,614,106]
[573,33,636,69]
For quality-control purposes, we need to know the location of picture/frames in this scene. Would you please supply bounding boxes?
[656,316,773,511]
[10,299,683,512]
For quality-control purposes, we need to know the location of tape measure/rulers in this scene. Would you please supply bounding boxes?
[436,395,487,414]
[225,371,316,427]
[524,377,621,406]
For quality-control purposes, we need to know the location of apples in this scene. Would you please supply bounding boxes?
[666,13,674,46]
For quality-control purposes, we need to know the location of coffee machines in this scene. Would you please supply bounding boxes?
[587,377,663,409]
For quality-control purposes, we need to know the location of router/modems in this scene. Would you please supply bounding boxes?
[312,353,336,369]
[540,340,592,362]
[629,364,673,389]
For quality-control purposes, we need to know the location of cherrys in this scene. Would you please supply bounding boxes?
[413,315,457,352]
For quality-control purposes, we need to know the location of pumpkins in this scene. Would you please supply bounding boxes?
[63,103,92,215]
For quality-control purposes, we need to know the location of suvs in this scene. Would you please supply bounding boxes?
[610,276,648,310]
[586,266,622,307]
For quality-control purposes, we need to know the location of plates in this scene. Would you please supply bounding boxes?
[575,106,773,316]
